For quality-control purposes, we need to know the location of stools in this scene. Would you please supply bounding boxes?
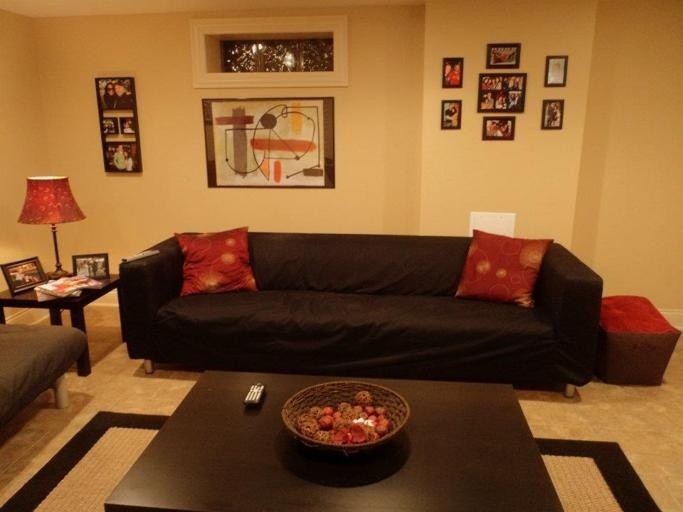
[600,294,682,386]
[1,321,88,450]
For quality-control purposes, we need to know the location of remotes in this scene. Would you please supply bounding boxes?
[122,250,160,264]
[243,382,264,405]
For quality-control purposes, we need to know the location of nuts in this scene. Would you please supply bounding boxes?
[296,390,392,445]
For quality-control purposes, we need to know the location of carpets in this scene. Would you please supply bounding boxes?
[1,409,665,512]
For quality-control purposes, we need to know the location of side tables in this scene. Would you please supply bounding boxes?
[0,271,122,376]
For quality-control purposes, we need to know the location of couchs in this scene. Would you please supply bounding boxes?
[116,231,604,399]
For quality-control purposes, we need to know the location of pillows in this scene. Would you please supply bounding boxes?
[173,225,260,297]
[455,229,554,309]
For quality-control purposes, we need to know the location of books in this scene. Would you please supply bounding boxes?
[34,273,104,302]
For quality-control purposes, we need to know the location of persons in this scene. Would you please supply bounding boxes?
[10,271,38,283]
[104,119,138,171]
[443,47,563,138]
[101,82,132,108]
[114,83,132,109]
[78,260,106,278]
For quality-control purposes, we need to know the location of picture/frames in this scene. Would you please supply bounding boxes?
[201,96,336,189]
[94,77,143,173]
[440,43,569,142]
[0,256,50,297]
[72,251,109,282]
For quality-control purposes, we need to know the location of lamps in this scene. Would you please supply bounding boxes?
[17,175,87,282]
